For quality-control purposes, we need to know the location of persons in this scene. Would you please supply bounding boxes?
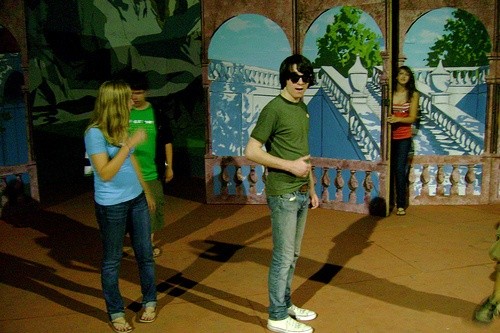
[129,72,173,256]
[245,54,318,333]
[385,66,418,216]
[84,80,158,333]
[474,222,500,322]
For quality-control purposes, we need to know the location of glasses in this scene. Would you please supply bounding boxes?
[132,90,145,95]
[288,72,313,84]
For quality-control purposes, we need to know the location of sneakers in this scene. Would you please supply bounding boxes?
[287,304,316,320]
[267,316,313,333]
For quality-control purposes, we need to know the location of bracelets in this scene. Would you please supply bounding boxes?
[165,162,171,169]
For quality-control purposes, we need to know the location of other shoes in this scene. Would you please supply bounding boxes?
[475,297,498,323]
[152,241,162,256]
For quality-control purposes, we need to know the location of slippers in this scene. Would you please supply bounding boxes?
[138,306,157,323]
[109,317,132,333]
[396,209,406,215]
